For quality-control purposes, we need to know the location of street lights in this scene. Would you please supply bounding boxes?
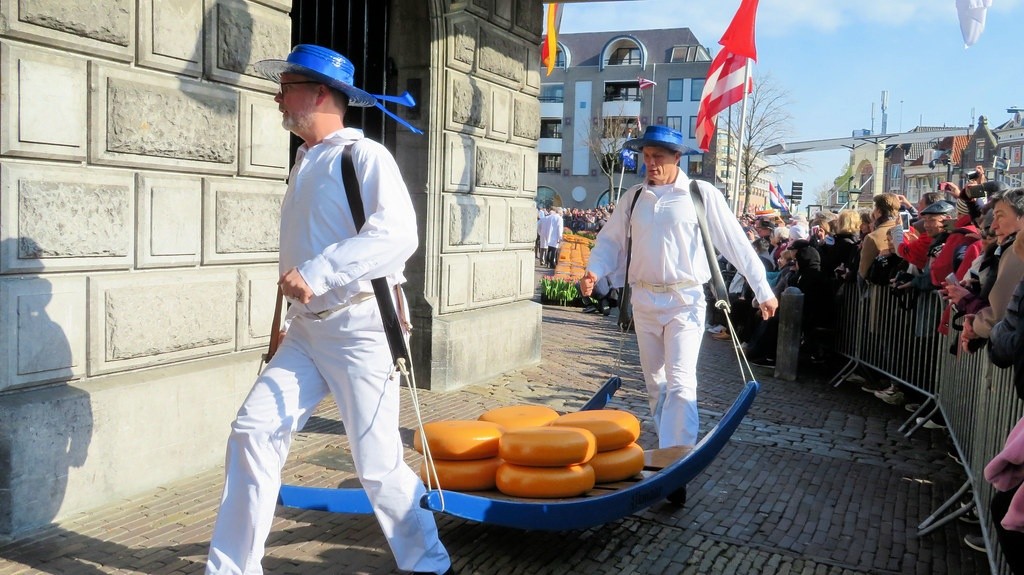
[929,154,953,201]
[900,101,904,133]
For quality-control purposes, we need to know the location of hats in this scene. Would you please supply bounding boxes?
[920,200,954,216]
[787,240,810,250]
[254,44,425,135]
[789,214,806,221]
[618,126,705,177]
[756,221,774,231]
[968,181,1010,199]
[957,188,988,230]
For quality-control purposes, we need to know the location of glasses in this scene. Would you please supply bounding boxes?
[279,81,333,96]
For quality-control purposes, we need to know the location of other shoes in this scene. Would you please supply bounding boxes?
[916,417,947,428]
[737,342,748,348]
[842,373,865,383]
[905,404,921,412]
[950,500,980,523]
[603,304,610,315]
[964,534,986,552]
[874,383,898,398]
[583,306,597,312]
[666,484,686,504]
[707,322,732,341]
[862,385,880,392]
[752,359,776,368]
[882,392,904,404]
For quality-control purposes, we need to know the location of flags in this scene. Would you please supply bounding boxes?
[695,1,758,154]
[543,2,563,77]
[638,77,657,90]
[766,180,790,214]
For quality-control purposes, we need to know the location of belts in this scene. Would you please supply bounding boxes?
[635,280,696,293]
[313,293,376,319]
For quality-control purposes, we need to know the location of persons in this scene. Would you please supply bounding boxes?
[579,125,779,501]
[203,44,451,575]
[538,165,1024,575]
